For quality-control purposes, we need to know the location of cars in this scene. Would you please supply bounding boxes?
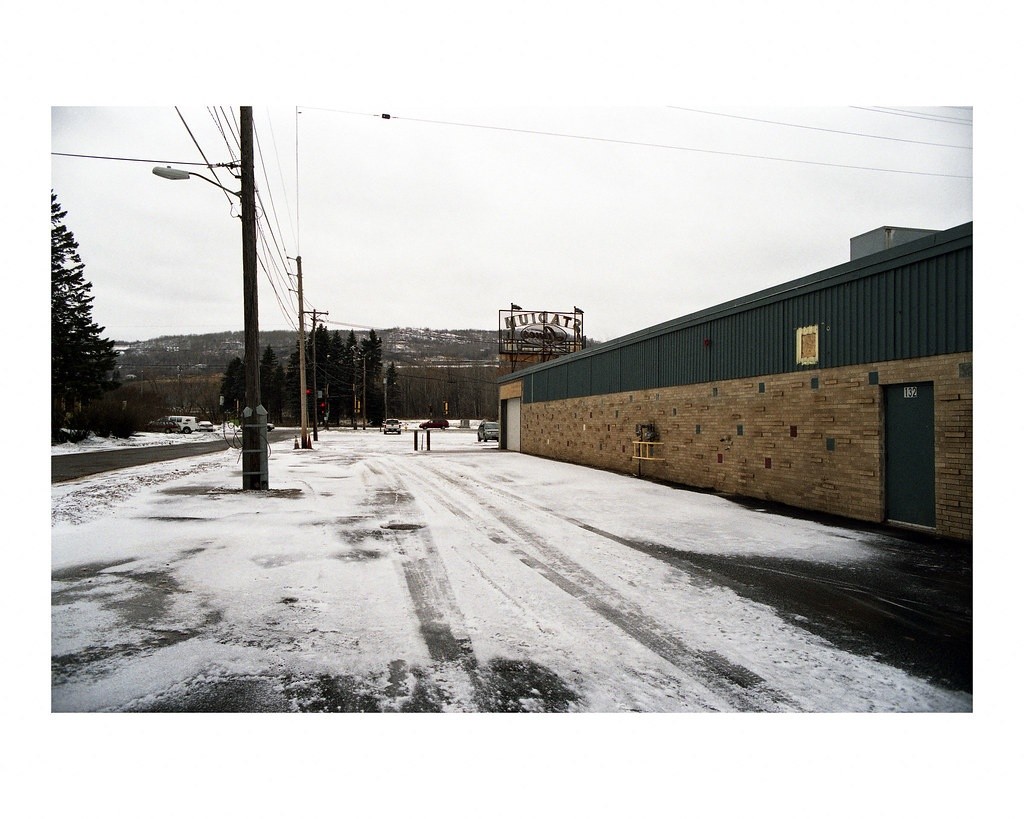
[477,422,499,442]
[195,421,213,432]
[240,421,275,432]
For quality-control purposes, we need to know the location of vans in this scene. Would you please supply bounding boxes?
[145,416,196,435]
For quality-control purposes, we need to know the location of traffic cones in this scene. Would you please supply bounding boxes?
[307,435,312,449]
[293,435,300,449]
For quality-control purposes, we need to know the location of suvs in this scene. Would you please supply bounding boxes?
[383,418,402,434]
[419,420,450,431]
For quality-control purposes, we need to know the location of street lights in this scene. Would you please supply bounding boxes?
[152,166,267,489]
